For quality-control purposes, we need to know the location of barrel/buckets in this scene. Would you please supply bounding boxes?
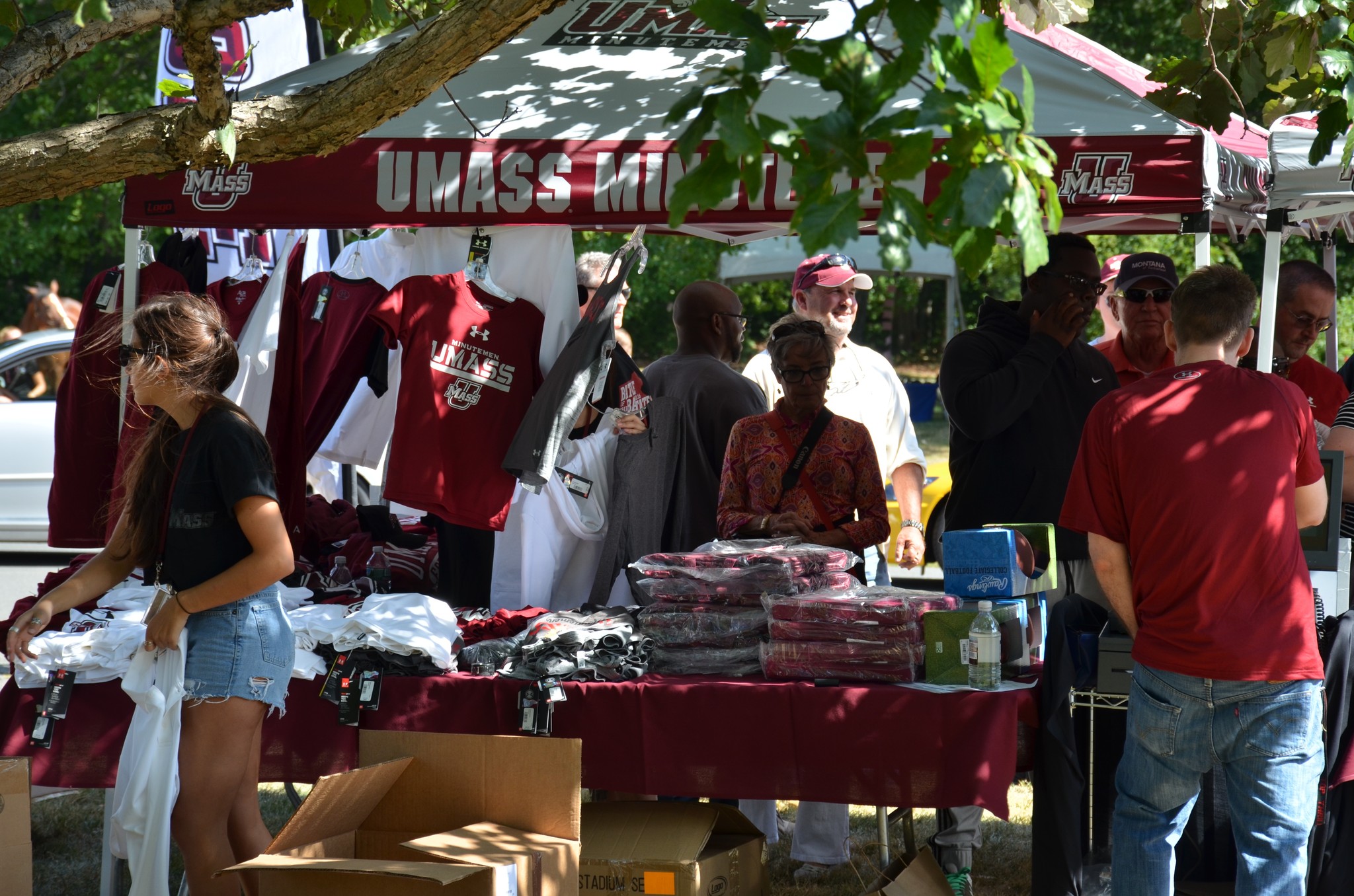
[905,381,939,422]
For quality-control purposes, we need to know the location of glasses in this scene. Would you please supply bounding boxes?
[1047,270,1106,298]
[1100,293,1116,308]
[116,342,149,368]
[776,358,832,384]
[800,254,859,289]
[1238,357,1293,377]
[583,286,632,299]
[1277,300,1333,333]
[771,321,826,349]
[1115,286,1177,305]
[709,312,748,329]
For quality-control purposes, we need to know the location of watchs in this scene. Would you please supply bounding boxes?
[900,520,924,538]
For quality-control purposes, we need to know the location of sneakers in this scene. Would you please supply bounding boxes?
[784,861,831,884]
[938,865,974,896]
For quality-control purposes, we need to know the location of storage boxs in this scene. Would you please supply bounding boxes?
[904,383,939,422]
[581,799,767,896]
[207,729,583,896]
[924,591,1048,684]
[1098,634,1134,693]
[942,524,1057,597]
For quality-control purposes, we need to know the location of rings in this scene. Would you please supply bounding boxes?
[8,626,21,634]
[144,640,155,646]
[915,556,920,562]
[30,616,42,625]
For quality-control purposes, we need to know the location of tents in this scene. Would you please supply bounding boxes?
[98,0,1268,896]
[1254,109,1354,377]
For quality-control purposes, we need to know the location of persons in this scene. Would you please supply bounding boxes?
[928,225,1354,896]
[6,292,296,896]
[557,400,646,612]
[742,252,928,573]
[716,318,891,884]
[586,281,767,808]
[568,252,637,364]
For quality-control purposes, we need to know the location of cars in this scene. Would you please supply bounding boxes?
[1,328,109,557]
[878,459,953,570]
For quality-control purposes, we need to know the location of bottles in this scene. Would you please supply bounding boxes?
[367,546,392,594]
[330,555,351,586]
[968,600,1001,692]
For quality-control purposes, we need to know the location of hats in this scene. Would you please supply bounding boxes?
[791,254,873,296]
[1114,251,1180,295]
[1099,252,1134,282]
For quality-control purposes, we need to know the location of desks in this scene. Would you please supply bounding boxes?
[0,660,1045,896]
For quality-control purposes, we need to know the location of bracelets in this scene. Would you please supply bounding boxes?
[760,513,774,531]
[176,590,195,614]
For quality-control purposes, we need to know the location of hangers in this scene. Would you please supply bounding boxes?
[609,224,648,274]
[117,226,156,270]
[225,229,264,287]
[465,235,516,302]
[333,251,365,279]
[286,229,308,244]
[182,227,200,243]
